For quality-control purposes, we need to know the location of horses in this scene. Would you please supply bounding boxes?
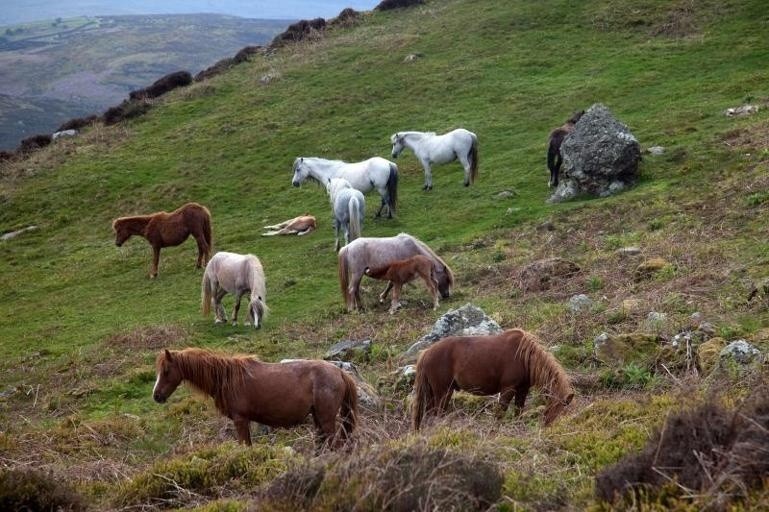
[260,211,317,236]
[152,347,358,453]
[410,327,576,431]
[546,109,586,189]
[337,233,455,315]
[326,176,366,253]
[201,251,267,330]
[390,128,479,192]
[112,202,212,281]
[292,156,399,221]
[364,254,439,315]
